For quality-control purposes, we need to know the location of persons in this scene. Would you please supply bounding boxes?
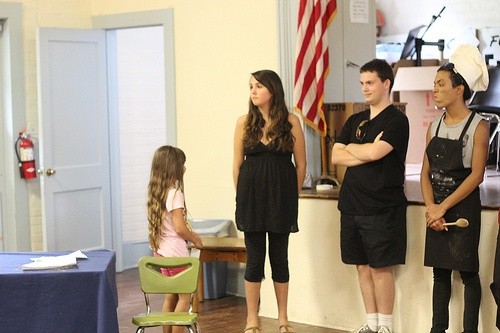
[147,146,203,333]
[421,43,489,333]
[233,70,307,333]
[331,63,409,333]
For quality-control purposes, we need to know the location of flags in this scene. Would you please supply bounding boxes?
[294,1,327,137]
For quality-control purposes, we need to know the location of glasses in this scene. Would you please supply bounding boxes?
[356,120,369,144]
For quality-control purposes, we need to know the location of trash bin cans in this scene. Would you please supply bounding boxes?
[188,219,232,300]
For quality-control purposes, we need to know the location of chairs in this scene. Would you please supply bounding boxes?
[132,255,201,333]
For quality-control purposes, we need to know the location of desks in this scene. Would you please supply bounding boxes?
[0,249,120,333]
[187,236,247,303]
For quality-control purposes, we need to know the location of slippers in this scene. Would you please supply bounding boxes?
[244,327,260,333]
[279,324,296,333]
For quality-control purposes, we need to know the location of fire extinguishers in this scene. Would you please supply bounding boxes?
[15,128,38,179]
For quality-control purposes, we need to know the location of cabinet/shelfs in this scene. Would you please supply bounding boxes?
[324,0,377,103]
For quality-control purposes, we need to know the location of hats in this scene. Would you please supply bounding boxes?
[449,42,489,91]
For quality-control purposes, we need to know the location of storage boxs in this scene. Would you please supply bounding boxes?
[392,58,447,164]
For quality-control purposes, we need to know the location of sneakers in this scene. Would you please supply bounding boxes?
[354,324,378,333]
[377,325,391,333]
